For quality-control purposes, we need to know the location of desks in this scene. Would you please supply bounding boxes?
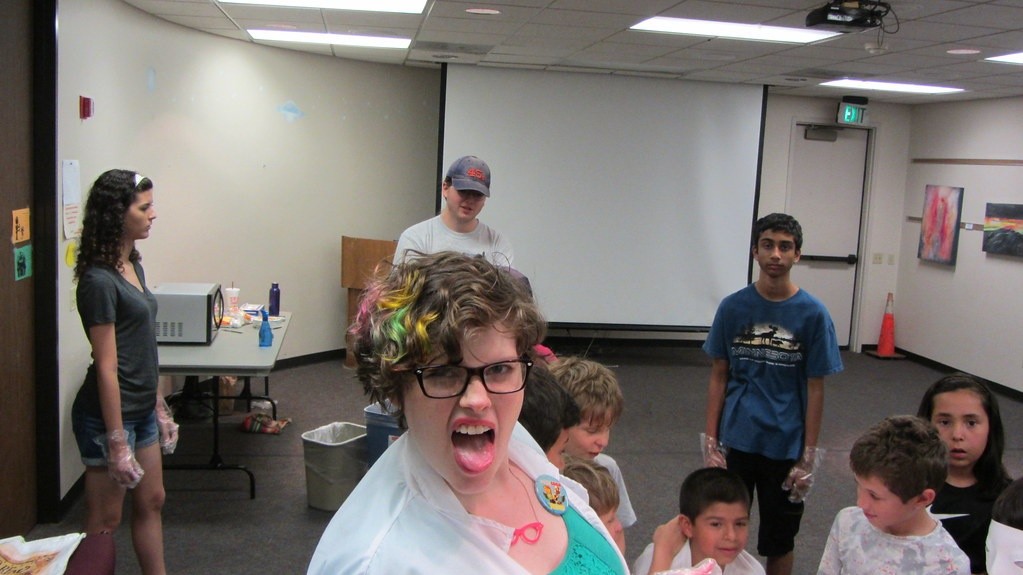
[149,311,292,502]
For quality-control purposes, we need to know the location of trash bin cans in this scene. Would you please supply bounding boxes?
[363,398,408,468]
[301,421,368,511]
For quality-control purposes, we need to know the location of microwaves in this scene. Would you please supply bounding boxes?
[150,282,224,346]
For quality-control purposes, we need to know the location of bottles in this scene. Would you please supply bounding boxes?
[269,282,280,316]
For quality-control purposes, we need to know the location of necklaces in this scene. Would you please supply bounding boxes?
[455,467,543,546]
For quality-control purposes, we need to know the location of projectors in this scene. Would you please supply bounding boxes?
[805,4,883,34]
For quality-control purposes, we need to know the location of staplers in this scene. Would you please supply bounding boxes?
[243,310,259,316]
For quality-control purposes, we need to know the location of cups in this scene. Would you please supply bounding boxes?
[225,287,240,313]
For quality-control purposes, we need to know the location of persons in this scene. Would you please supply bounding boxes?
[393,154,514,269]
[917,371,1012,575]
[304,249,724,575]
[702,211,844,575]
[817,412,972,575]
[69,169,178,575]
[984,474,1023,575]
[497,267,638,557]
[633,466,767,575]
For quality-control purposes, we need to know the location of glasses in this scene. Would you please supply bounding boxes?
[399,352,533,399]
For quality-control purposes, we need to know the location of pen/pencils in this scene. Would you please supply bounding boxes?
[271,327,282,330]
[224,330,243,334]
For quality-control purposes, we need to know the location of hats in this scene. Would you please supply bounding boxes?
[446,155,491,197]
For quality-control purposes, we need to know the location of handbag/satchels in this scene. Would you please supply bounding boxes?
[217,376,237,416]
